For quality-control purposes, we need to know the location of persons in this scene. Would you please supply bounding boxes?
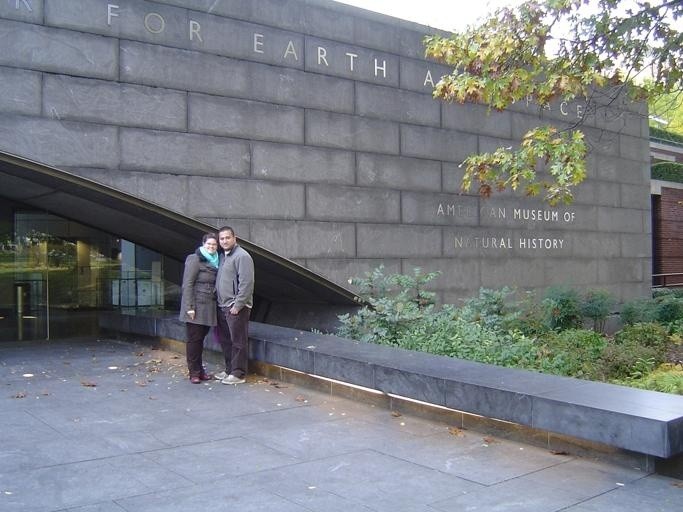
[177,232,220,384]
[214,225,256,385]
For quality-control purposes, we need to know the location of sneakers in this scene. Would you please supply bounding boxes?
[222,375,246,384]
[200,372,212,380]
[214,371,228,380]
[191,377,201,383]
